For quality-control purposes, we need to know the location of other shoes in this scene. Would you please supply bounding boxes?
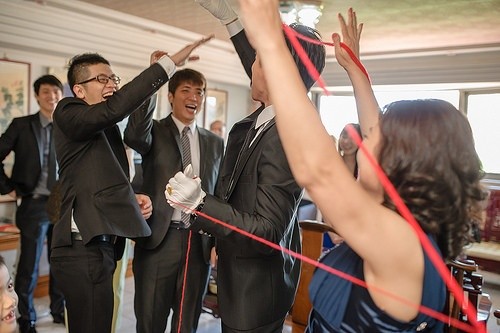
[19,318,37,333]
[53,314,64,322]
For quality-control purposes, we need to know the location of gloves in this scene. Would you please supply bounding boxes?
[198,0,238,24]
[164,164,206,215]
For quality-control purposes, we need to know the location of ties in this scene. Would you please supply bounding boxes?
[180,126,193,228]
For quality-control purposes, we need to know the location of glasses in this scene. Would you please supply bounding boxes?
[78,74,121,84]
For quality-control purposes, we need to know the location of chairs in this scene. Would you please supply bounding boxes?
[462,189,500,276]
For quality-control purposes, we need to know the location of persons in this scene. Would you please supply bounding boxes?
[124,52,228,333]
[234,0,489,333]
[164,0,325,333]
[50,34,215,333]
[0,74,65,333]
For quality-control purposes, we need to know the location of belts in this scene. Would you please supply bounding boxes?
[22,193,48,201]
[85,233,122,245]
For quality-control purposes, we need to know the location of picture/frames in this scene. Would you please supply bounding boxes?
[202,88,228,140]
[0,59,31,132]
